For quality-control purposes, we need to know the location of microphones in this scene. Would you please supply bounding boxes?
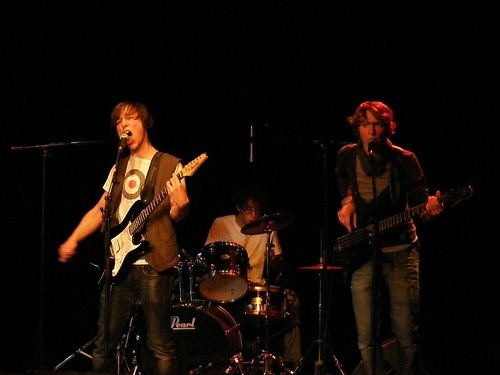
[118,133,129,152]
[368,139,376,153]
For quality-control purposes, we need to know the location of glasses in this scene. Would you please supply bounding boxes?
[242,207,261,216]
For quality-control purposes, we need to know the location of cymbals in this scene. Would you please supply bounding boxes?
[296,262,347,275]
[239,212,295,236]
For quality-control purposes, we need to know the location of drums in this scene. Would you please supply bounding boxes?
[197,239,251,303]
[165,258,207,298]
[132,299,243,375]
[238,285,297,324]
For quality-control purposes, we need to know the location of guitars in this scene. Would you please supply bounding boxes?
[318,186,475,266]
[103,151,209,287]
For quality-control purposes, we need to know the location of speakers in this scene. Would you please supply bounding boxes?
[349,331,403,375]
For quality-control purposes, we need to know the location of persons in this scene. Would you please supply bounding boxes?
[196,176,302,375]
[335,99,443,375]
[57,101,190,375]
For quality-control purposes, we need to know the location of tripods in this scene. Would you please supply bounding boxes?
[284,271,346,375]
[240,228,297,374]
